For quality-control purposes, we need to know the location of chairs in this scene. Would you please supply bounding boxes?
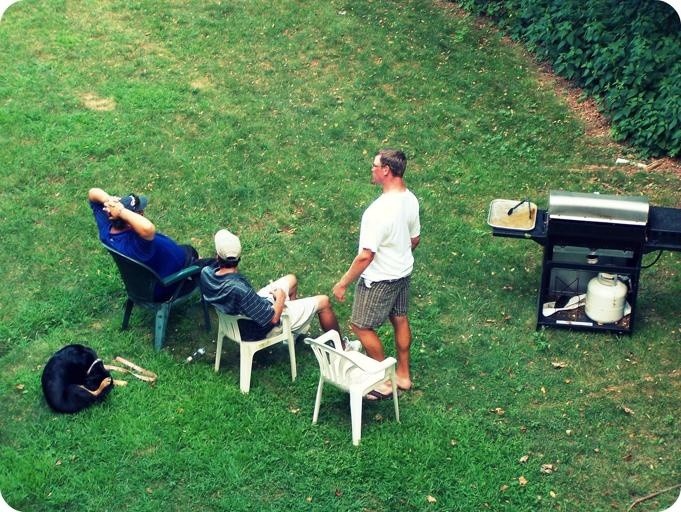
[99,242,210,352]
[214,309,296,395]
[303,329,399,446]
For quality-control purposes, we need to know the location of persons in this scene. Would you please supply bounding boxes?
[333,148,421,401]
[88,187,200,302]
[200,229,346,350]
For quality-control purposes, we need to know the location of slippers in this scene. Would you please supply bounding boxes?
[398,383,413,391]
[363,389,402,402]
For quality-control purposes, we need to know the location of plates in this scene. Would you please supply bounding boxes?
[487,198,537,230]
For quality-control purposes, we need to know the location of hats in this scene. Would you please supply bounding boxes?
[215,229,242,261]
[108,193,147,224]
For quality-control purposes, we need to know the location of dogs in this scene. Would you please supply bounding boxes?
[41,343,114,413]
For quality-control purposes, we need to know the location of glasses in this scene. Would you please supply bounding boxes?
[372,164,386,169]
[137,209,144,216]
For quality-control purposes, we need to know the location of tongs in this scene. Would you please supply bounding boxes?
[507,196,533,219]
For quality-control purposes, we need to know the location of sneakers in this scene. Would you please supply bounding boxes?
[343,336,362,353]
[283,325,311,344]
